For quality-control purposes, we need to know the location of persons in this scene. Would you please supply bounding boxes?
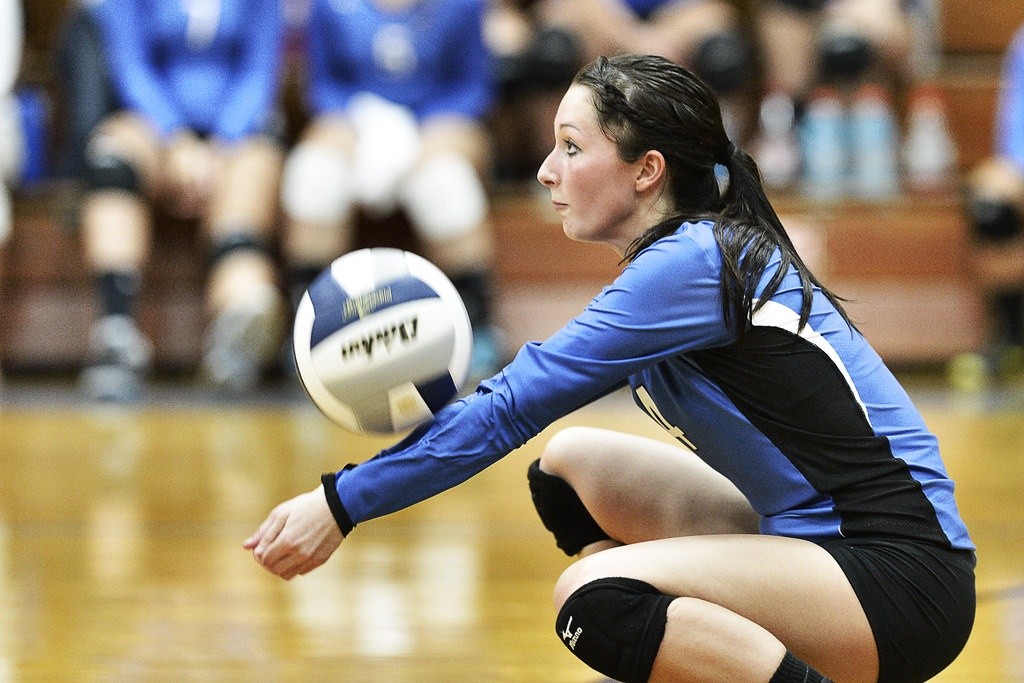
[2,0,1024,397]
[249,51,978,681]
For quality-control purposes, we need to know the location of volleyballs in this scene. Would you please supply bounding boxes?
[292,246,477,439]
